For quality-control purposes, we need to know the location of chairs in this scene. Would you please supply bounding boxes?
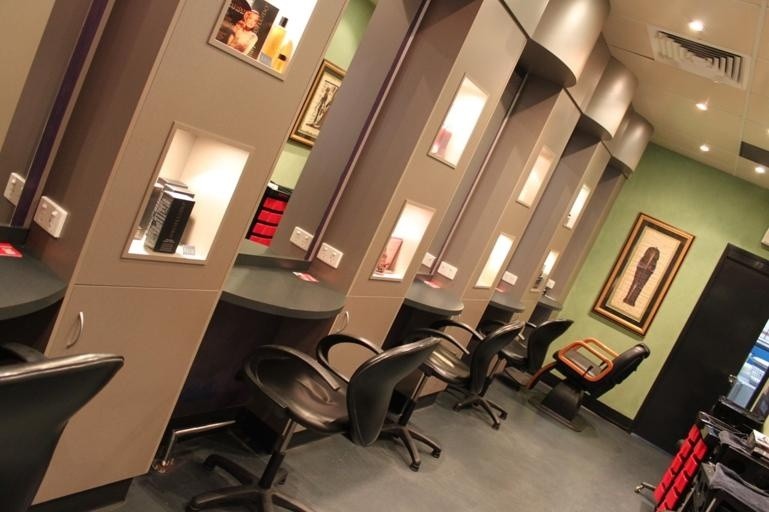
[183,334,442,511]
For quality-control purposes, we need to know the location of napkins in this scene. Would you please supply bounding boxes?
[427,169,556,284]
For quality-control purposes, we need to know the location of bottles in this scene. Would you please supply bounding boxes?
[257,16,293,74]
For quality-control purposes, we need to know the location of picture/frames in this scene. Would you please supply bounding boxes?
[590,212,695,337]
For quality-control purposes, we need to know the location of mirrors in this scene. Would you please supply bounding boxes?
[1,0,114,245]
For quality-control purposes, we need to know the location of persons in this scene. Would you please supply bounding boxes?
[226,10,260,54]
